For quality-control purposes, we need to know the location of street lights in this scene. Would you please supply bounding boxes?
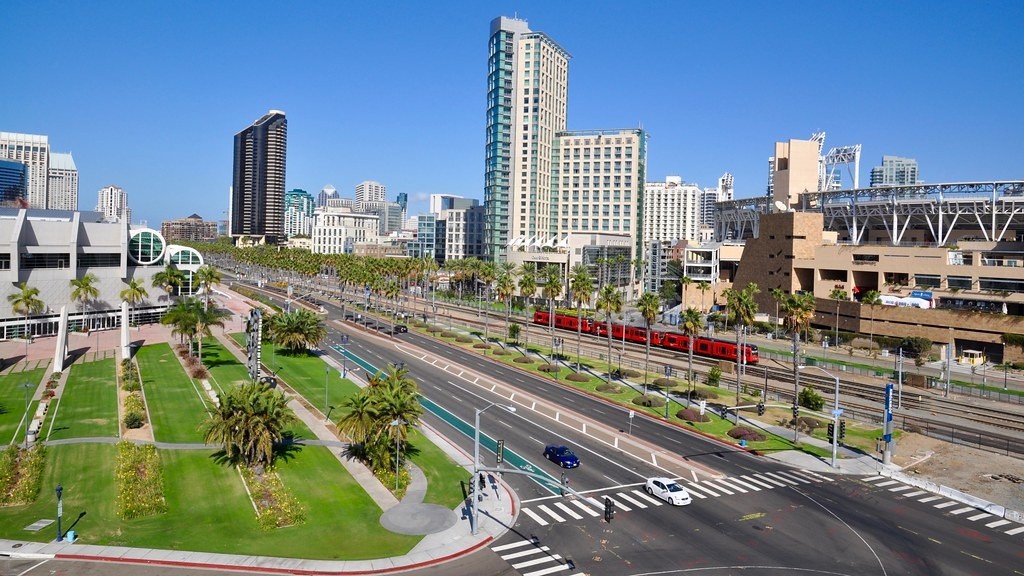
[553,336,561,380]
[664,366,673,419]
[55,482,63,541]
[944,328,954,397]
[340,333,349,379]
[471,403,516,535]
[288,286,313,315]
[1002,341,1007,391]
[797,366,839,468]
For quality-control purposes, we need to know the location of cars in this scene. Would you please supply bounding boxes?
[544,444,580,469]
[646,477,692,506]
[393,325,408,333]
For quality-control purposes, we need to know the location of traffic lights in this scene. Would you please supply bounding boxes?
[840,420,845,438]
[758,402,764,416]
[793,404,799,419]
[721,404,728,420]
[828,423,833,437]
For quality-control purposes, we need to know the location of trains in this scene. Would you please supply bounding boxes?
[534,310,760,364]
